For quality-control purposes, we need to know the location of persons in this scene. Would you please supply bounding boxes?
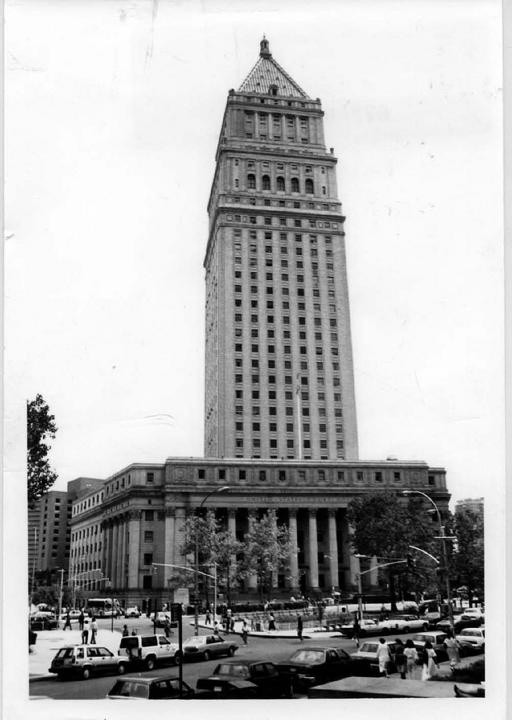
[203,600,235,636]
[77,612,84,630]
[161,604,168,611]
[353,620,361,648]
[421,641,437,679]
[394,638,407,679]
[402,640,419,676]
[90,617,97,644]
[123,625,129,636]
[164,615,174,637]
[339,607,347,620]
[181,603,186,614]
[297,617,304,641]
[442,631,459,668]
[376,638,391,677]
[242,622,249,644]
[379,607,388,622]
[268,612,275,631]
[82,620,90,644]
[62,612,73,630]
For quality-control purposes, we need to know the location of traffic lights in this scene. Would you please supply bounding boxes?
[154,568,158,575]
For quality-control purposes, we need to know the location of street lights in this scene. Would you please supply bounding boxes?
[401,490,455,638]
[57,569,65,622]
[324,555,335,593]
[195,486,232,636]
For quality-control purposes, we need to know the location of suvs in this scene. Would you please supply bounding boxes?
[117,633,185,672]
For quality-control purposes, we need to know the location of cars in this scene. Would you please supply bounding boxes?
[196,659,286,700]
[274,647,372,694]
[150,611,178,628]
[351,640,399,666]
[182,635,240,663]
[339,600,485,656]
[30,602,139,631]
[48,643,130,680]
[104,671,197,700]
